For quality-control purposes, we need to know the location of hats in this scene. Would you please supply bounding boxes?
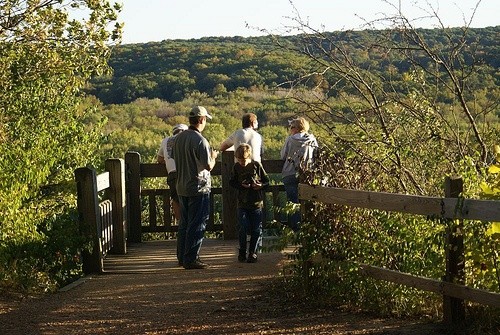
[172,124,188,132]
[188,105,212,120]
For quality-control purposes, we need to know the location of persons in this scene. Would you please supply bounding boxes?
[280,118,318,238]
[157,124,189,220]
[221,113,270,263]
[171,106,219,269]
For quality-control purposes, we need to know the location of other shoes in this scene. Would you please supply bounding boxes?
[237,248,247,262]
[179,257,203,267]
[247,253,257,263]
[182,260,208,270]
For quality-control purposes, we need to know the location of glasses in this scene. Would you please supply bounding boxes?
[290,126,295,129]
